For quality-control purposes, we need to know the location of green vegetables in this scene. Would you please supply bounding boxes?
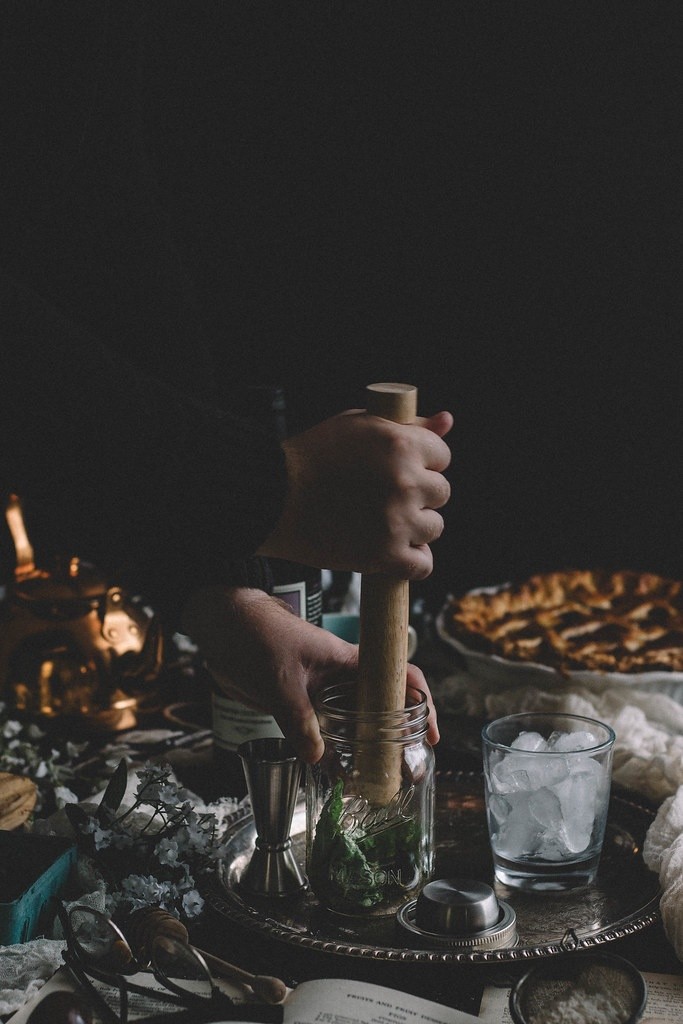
[311,776,425,908]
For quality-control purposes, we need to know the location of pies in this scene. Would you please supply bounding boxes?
[444,570,683,671]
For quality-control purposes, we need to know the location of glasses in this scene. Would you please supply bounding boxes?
[49,894,235,1024]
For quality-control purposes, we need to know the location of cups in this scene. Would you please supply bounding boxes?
[481,710,615,894]
[322,610,418,663]
[236,737,307,899]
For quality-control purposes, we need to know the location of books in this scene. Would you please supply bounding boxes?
[4,964,484,1024]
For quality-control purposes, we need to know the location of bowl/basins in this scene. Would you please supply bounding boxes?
[435,582,683,705]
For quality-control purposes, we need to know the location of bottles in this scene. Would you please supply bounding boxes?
[210,554,322,759]
[302,678,437,918]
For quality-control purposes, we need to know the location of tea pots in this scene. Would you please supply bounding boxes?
[1,483,173,734]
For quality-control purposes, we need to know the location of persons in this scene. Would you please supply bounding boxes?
[0,271,455,786]
